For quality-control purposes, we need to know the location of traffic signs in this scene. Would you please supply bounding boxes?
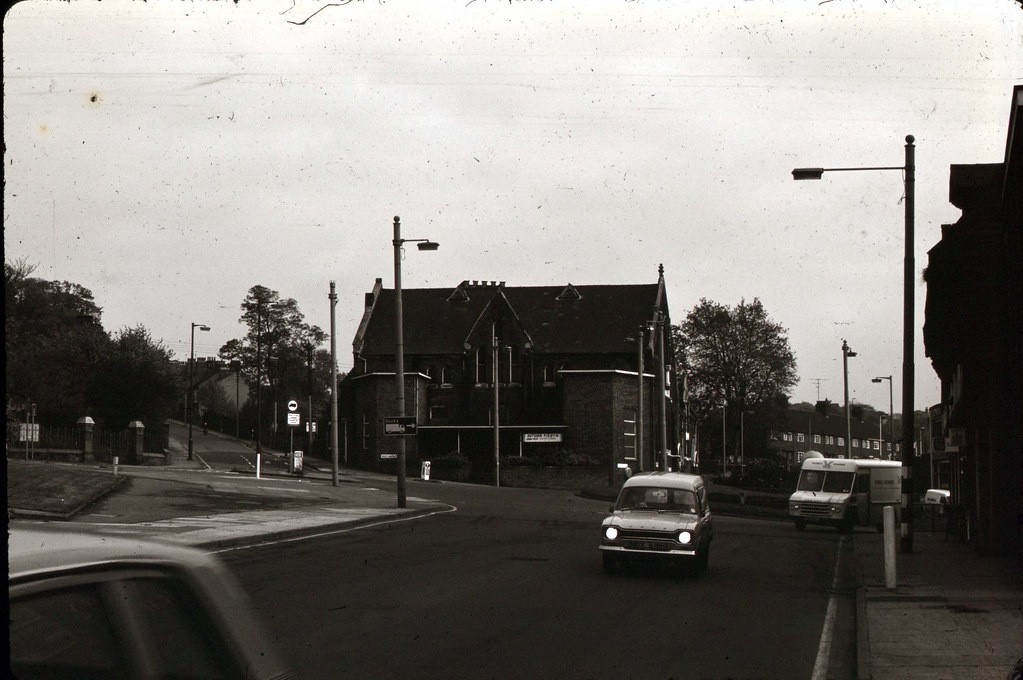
[383,417,418,435]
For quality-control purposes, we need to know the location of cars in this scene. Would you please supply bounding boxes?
[10,525,293,680]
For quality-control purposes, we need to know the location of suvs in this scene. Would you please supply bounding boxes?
[598,471,714,582]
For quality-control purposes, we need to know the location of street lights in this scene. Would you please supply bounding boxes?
[187,322,211,458]
[392,215,440,509]
[869,374,895,462]
[791,134,915,570]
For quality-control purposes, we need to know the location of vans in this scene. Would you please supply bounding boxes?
[789,459,880,534]
[922,488,951,518]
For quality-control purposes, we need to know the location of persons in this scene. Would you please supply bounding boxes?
[939,496,946,514]
